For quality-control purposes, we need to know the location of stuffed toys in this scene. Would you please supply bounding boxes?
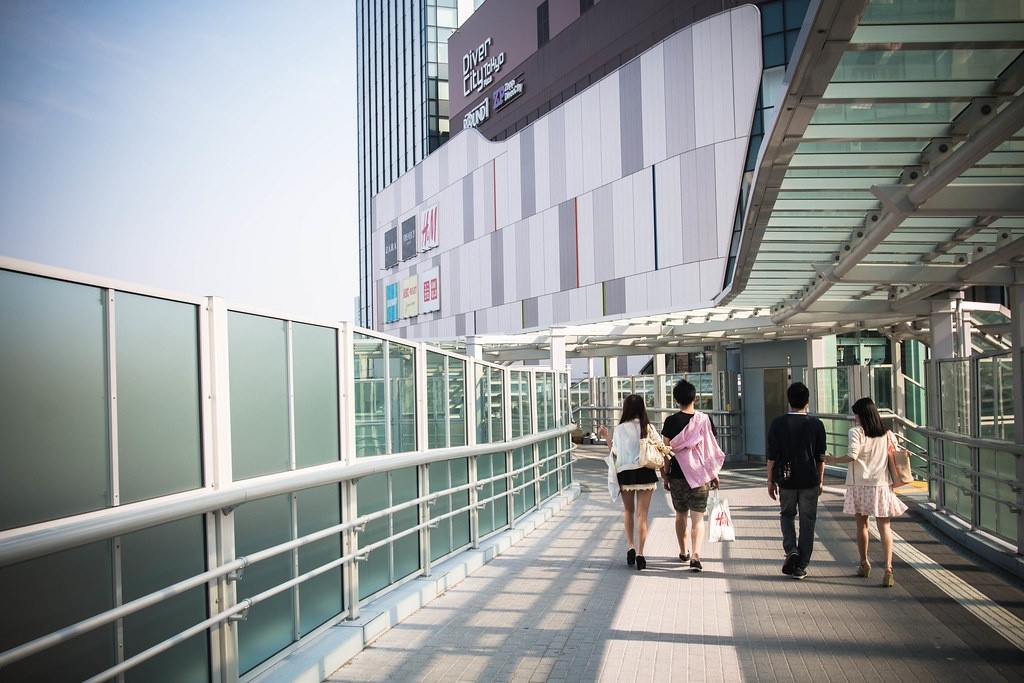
[657,441,676,460]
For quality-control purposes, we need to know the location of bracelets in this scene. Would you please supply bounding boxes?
[767,481,773,482]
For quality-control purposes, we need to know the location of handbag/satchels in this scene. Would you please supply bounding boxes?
[774,457,791,486]
[605,447,621,503]
[888,431,915,488]
[707,487,736,543]
[639,424,665,469]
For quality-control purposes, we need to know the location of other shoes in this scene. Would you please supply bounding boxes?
[689,559,703,572]
[792,568,808,580]
[781,550,800,575]
[678,550,690,563]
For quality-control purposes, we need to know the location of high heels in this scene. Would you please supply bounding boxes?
[636,556,647,570]
[883,564,895,587]
[626,549,636,567]
[856,559,871,578]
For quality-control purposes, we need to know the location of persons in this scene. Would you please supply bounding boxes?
[661,379,721,572]
[820,397,909,586]
[598,394,670,571]
[767,381,828,579]
